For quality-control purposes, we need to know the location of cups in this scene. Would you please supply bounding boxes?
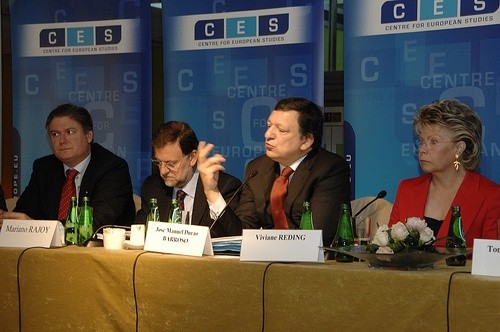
[352,216,371,248]
[130,225,145,245]
[102,227,126,249]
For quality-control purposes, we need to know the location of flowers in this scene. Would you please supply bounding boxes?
[367,214,436,255]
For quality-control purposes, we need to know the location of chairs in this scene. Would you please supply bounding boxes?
[347,197,392,240]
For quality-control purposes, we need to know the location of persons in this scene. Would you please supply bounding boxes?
[1,103,135,235]
[134,120,243,239]
[197,98,352,249]
[388,99,500,249]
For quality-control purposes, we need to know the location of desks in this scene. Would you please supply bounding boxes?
[0,237,500,332]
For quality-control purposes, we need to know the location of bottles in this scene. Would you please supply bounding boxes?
[77,196,94,246]
[335,203,355,263]
[145,198,160,239]
[445,205,467,265]
[168,199,182,224]
[298,201,316,230]
[64,196,79,245]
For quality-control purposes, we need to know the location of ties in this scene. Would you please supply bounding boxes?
[270,166,293,230]
[58,169,79,221]
[176,190,187,222]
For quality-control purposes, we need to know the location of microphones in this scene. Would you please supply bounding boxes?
[325,190,388,260]
[209,169,258,231]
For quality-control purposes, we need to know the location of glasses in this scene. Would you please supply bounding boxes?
[414,136,459,149]
[152,155,185,168]
